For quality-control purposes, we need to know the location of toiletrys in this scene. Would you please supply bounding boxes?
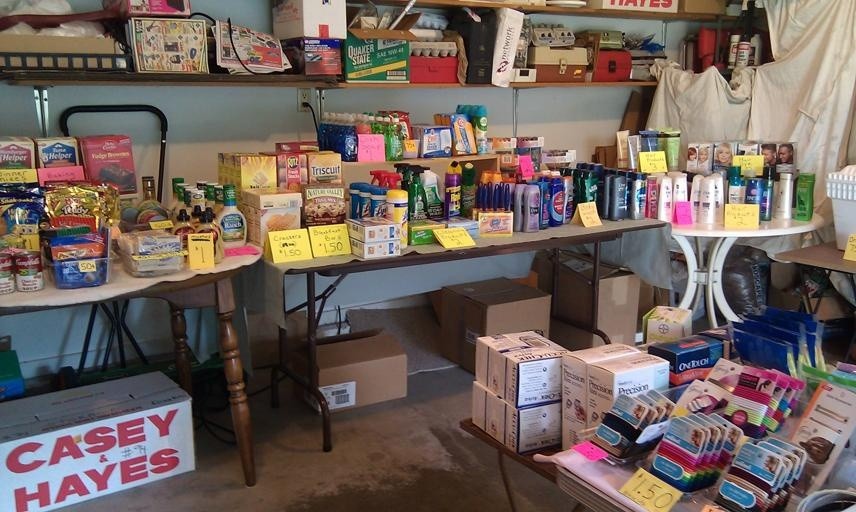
[317,110,816,249]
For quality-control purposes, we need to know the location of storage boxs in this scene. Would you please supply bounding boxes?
[822,162,856,252]
[0,33,116,71]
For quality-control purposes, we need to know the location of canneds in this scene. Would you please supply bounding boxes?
[14,251,45,292]
[0,253,14,294]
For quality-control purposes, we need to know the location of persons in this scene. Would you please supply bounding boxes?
[714,143,733,167]
[760,145,777,167]
[698,147,711,174]
[688,147,697,162]
[778,144,794,165]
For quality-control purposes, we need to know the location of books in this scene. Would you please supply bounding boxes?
[124,23,129,46]
[211,26,293,75]
[215,19,284,72]
[130,17,210,74]
[127,20,136,72]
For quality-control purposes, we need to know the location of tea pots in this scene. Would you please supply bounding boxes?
[679,33,700,73]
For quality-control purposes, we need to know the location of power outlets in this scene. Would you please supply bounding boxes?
[296,87,312,114]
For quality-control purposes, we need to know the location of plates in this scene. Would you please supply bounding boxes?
[546,2,587,8]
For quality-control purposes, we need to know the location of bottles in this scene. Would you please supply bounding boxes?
[348,180,407,249]
[456,103,490,154]
[316,111,403,163]
[136,174,248,265]
[760,166,775,222]
[728,32,762,67]
[443,158,477,219]
